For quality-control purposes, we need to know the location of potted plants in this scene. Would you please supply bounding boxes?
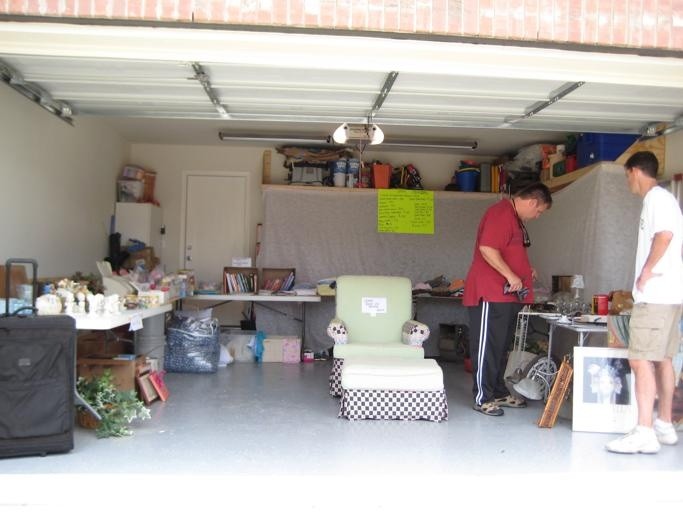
[75,366,152,440]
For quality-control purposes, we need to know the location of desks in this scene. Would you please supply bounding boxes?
[34,292,321,363]
[542,318,607,407]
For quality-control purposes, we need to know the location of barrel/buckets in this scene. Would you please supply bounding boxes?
[454,168,481,191]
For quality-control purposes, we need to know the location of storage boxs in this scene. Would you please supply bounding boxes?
[117,180,144,203]
[75,332,145,398]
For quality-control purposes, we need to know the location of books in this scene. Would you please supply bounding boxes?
[226,271,294,293]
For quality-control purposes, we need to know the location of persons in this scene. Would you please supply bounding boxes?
[605,152,683,455]
[463,182,553,416]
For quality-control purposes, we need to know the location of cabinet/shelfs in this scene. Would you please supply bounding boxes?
[222,266,295,295]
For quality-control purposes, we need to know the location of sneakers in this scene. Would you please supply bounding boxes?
[494,394,527,408]
[473,402,504,416]
[606,425,661,454]
[653,418,678,445]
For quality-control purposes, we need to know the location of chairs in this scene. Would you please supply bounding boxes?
[326,274,448,423]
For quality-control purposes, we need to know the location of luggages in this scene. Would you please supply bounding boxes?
[0,258,75,459]
